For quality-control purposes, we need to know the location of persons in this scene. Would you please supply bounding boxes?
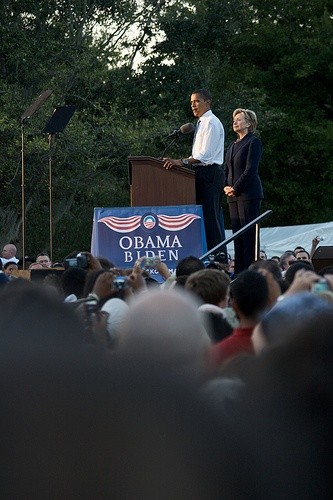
[223,108,264,279]
[0,234,333,500]
[162,88,228,265]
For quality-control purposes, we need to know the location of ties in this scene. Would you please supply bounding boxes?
[193,121,201,144]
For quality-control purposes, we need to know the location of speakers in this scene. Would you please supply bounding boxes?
[312,246,333,273]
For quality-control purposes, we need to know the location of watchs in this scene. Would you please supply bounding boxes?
[181,158,189,167]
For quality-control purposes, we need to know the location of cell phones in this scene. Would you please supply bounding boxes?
[83,302,101,326]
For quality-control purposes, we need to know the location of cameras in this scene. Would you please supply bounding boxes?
[311,283,331,293]
[113,280,128,290]
[144,257,159,268]
[64,255,87,270]
[317,236,325,241]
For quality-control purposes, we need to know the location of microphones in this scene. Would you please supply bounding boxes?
[167,123,195,139]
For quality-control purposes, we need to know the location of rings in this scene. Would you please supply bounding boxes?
[137,262,141,265]
[135,261,138,263]
